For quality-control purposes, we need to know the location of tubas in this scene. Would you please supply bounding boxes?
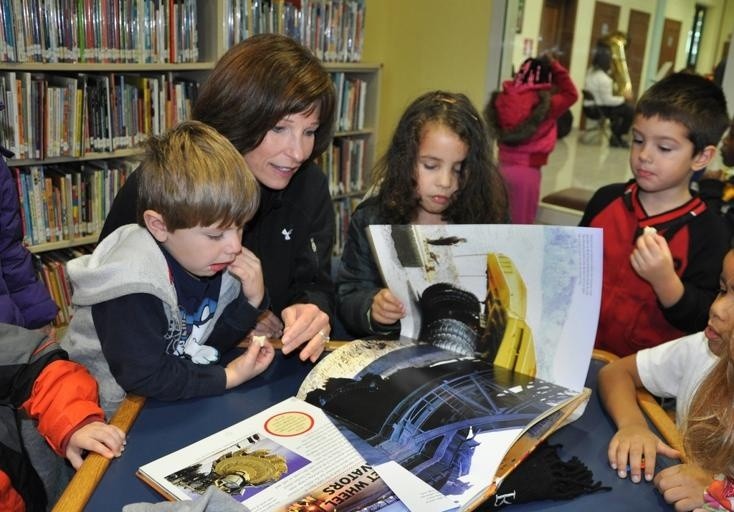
[598,32,632,97]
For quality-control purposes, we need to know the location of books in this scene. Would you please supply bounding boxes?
[134,222,604,512]
[1,1,197,326]
[314,138,366,196]
[331,71,366,132]
[229,1,367,63]
[338,200,362,255]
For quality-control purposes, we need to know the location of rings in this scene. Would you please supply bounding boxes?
[319,331,331,340]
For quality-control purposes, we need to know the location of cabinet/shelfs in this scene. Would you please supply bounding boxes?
[1,0,383,330]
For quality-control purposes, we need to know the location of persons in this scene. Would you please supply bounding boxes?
[335,91,512,345]
[65,121,278,428]
[681,333,734,512]
[0,321,127,512]
[488,49,578,222]
[596,244,734,512]
[720,118,733,219]
[577,71,734,358]
[581,43,635,149]
[95,33,337,364]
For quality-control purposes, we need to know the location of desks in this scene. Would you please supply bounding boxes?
[48,339,687,512]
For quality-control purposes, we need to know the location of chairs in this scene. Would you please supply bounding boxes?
[582,90,622,147]
